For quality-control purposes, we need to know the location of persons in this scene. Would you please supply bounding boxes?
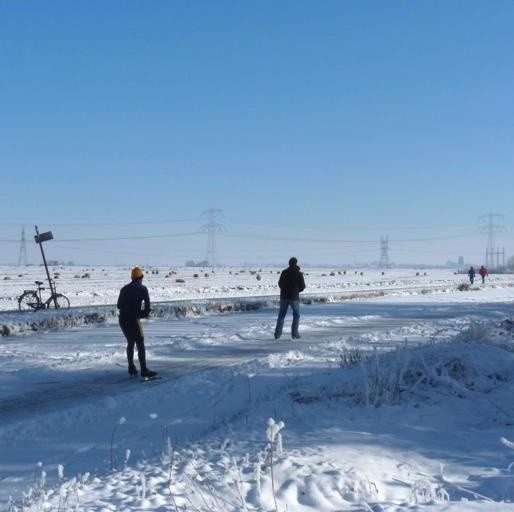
[468,266,476,285]
[116,267,157,378]
[479,265,488,284]
[272,257,306,339]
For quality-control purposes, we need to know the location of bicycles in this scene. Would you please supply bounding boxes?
[18,279,71,313]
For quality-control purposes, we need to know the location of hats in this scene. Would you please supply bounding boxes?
[131,267,143,278]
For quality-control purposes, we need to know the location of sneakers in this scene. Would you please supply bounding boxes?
[129,366,137,374]
[141,368,157,376]
